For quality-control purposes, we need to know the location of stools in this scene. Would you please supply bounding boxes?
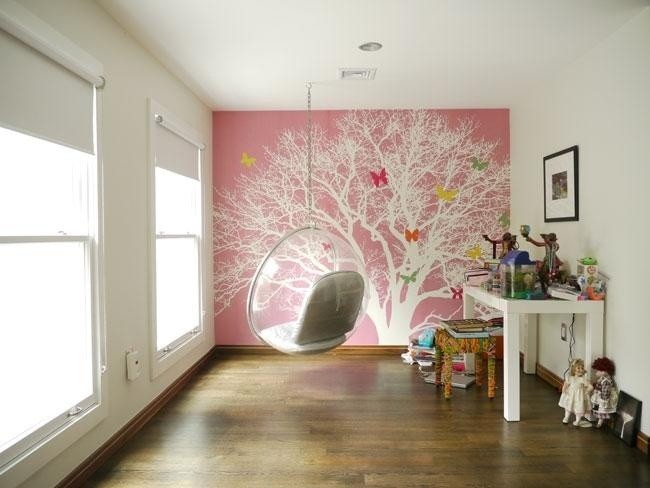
[435,328,498,400]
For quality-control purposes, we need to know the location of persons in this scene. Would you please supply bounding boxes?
[587,357,619,430]
[558,356,591,427]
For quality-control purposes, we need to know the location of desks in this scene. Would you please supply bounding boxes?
[463,279,605,422]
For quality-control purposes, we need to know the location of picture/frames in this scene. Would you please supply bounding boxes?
[543,143,580,222]
[610,389,643,446]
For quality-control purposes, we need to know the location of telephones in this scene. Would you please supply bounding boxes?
[547,287,580,301]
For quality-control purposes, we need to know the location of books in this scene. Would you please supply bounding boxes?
[406,315,490,390]
